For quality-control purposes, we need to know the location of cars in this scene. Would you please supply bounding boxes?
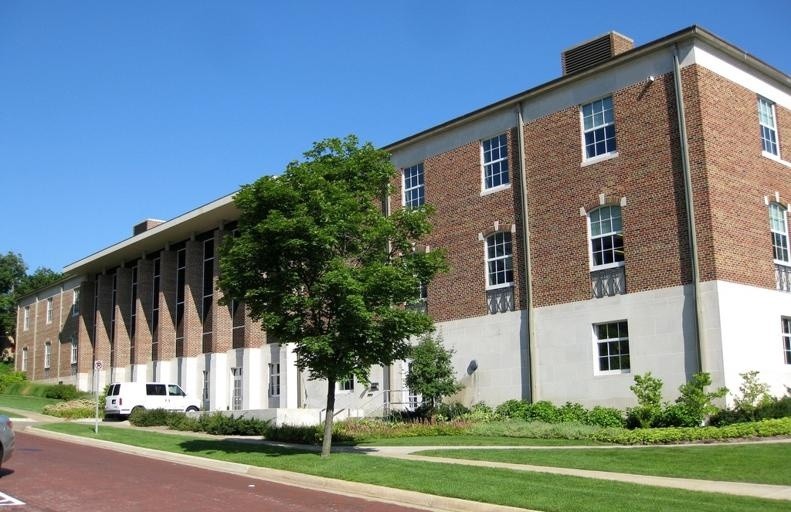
[0,410,15,479]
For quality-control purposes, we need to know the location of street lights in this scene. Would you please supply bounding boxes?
[95,359,101,433]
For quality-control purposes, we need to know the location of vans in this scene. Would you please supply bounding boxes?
[105,379,200,421]
